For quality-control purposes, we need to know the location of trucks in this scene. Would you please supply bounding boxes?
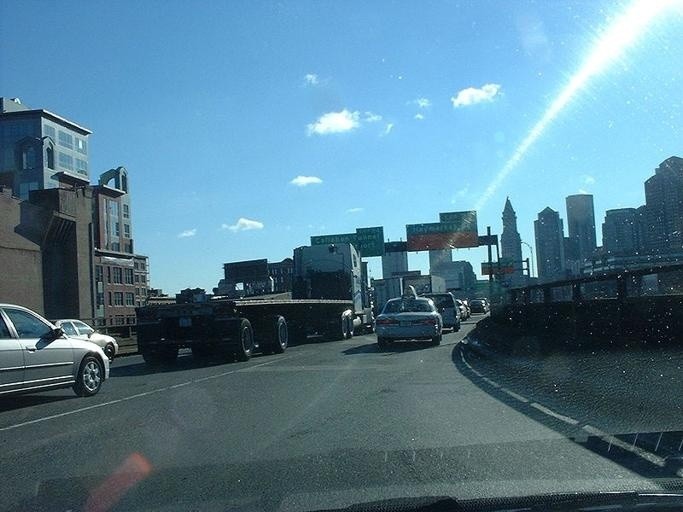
[134,241,375,365]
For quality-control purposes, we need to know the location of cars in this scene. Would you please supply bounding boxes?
[376,284,446,347]
[0,303,109,396]
[456,297,489,322]
[49,318,119,363]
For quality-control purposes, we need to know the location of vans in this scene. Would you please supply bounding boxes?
[420,292,461,332]
